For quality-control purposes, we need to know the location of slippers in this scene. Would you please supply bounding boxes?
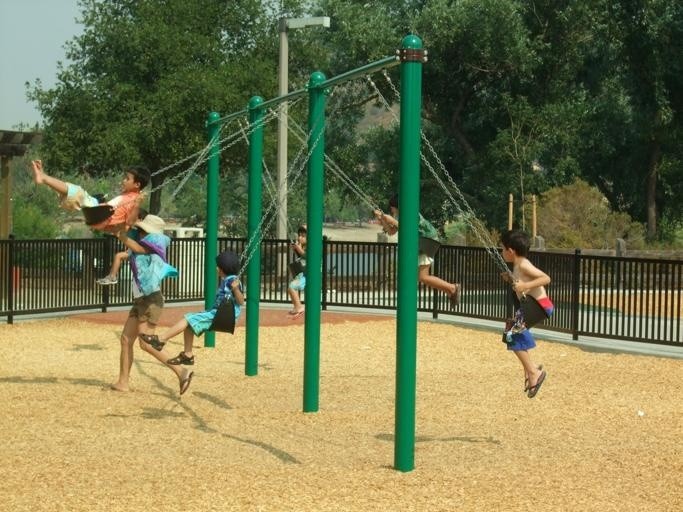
[180,372,193,394]
[524,365,546,397]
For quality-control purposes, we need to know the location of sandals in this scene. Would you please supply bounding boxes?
[138,333,164,351]
[167,352,194,365]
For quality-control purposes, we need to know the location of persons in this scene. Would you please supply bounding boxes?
[97,210,149,284]
[30,158,149,231]
[288,224,307,318]
[373,194,462,308]
[139,250,243,365]
[497,230,555,398]
[108,214,194,395]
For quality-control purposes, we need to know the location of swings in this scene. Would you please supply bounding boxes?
[129,121,233,253]
[369,69,547,329]
[269,100,442,261]
[237,116,308,279]
[207,83,351,334]
[80,97,304,224]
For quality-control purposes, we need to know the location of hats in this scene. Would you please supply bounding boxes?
[216,252,239,274]
[134,214,166,234]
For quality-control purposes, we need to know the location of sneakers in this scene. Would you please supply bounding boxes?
[96,274,119,286]
[289,308,305,320]
[447,283,461,308]
